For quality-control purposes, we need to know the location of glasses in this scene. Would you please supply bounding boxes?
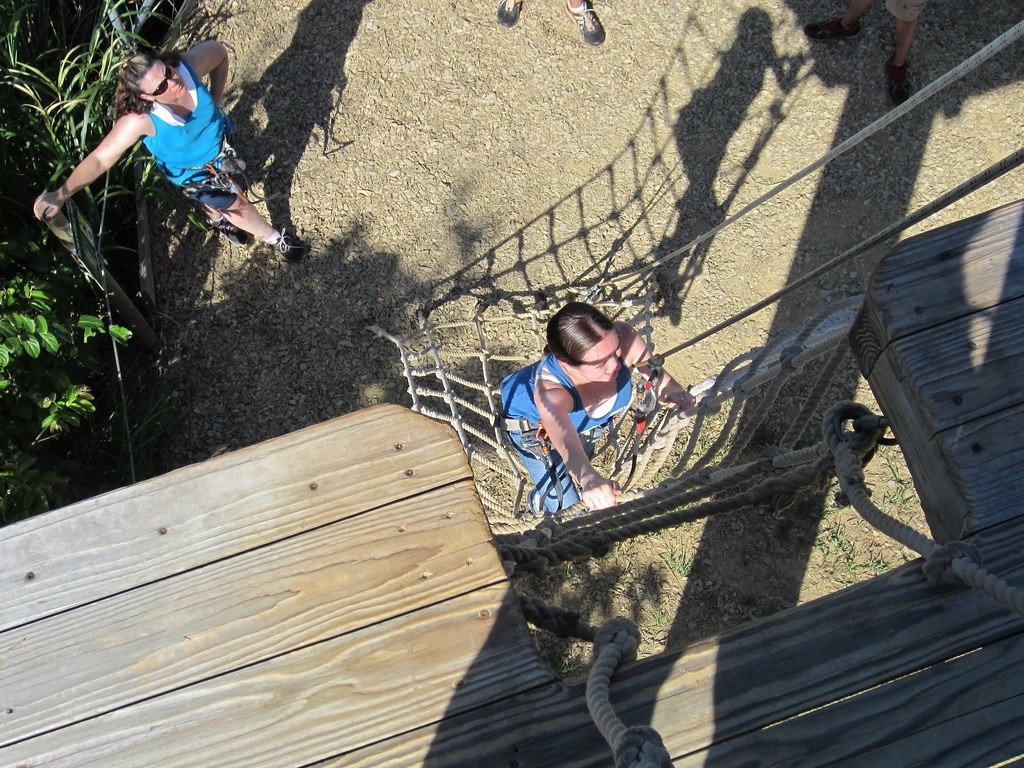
[144,58,172,97]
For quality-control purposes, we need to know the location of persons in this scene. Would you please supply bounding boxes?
[32,38,307,264]
[804,0,926,106]
[497,0,606,46]
[501,301,697,514]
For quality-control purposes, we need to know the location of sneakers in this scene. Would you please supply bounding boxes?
[267,228,305,262]
[564,0,605,47]
[885,54,910,105]
[207,216,249,247]
[497,0,523,27]
[804,19,864,39]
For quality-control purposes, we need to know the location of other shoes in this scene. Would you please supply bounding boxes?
[528,487,547,515]
